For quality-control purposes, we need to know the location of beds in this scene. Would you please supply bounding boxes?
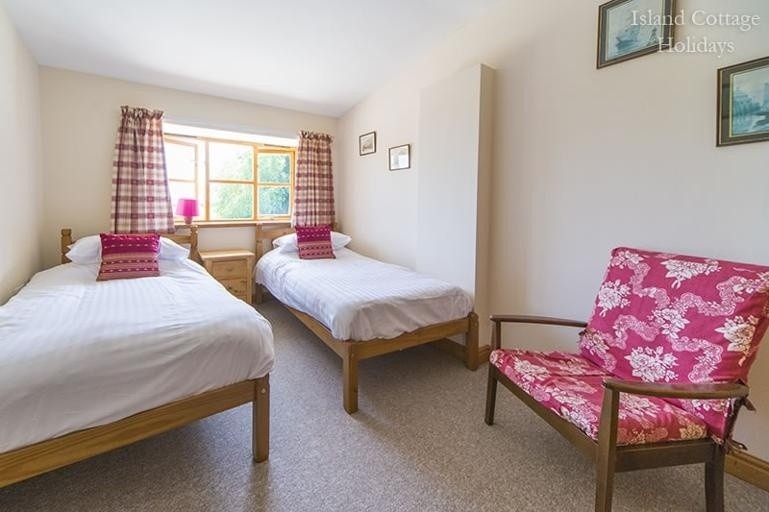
[256,221,482,415]
[1,225,277,490]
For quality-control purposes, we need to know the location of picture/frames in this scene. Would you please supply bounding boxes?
[594,1,679,71]
[713,55,769,149]
[386,143,412,172]
[358,130,377,158]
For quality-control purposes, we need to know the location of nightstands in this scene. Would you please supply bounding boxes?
[198,246,255,306]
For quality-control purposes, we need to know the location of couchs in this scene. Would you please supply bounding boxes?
[483,242,767,512]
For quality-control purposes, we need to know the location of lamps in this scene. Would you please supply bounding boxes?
[173,198,202,225]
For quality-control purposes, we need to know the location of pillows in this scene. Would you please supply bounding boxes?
[270,223,354,261]
[65,231,190,283]
